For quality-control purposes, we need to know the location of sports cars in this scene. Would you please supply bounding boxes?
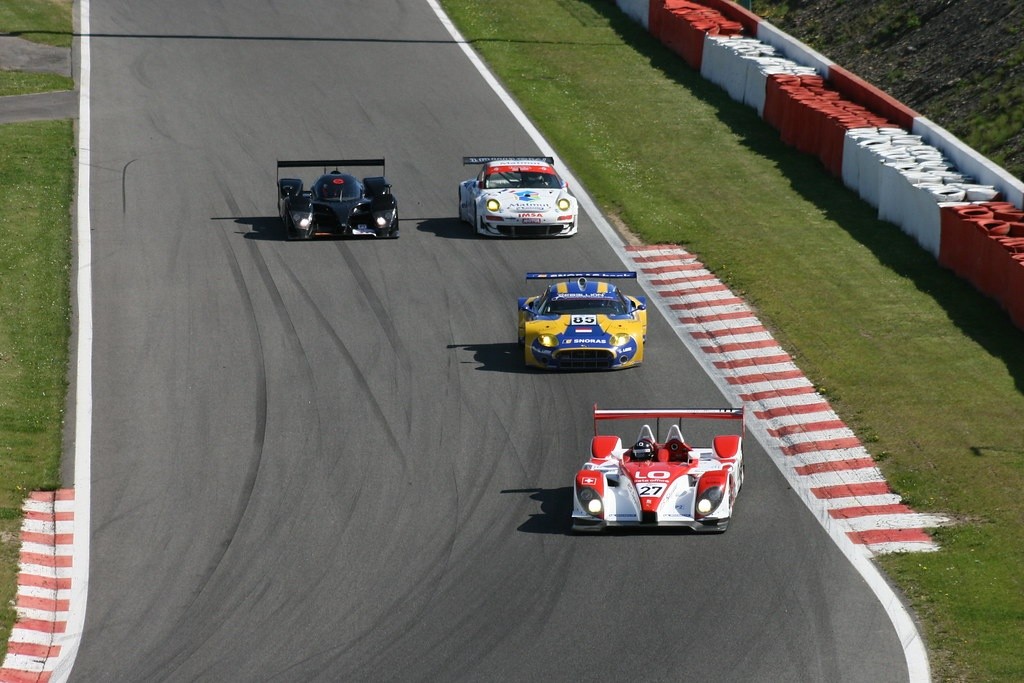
[517,272,648,372]
[276,158,400,241]
[458,155,579,238]
[569,405,748,536]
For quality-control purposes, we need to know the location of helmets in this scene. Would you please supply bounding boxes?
[528,172,544,182]
[632,440,654,460]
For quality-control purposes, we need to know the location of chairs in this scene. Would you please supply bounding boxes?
[631,424,659,461]
[662,425,693,462]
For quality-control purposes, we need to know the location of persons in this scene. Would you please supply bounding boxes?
[631,440,655,461]
[524,173,549,186]
